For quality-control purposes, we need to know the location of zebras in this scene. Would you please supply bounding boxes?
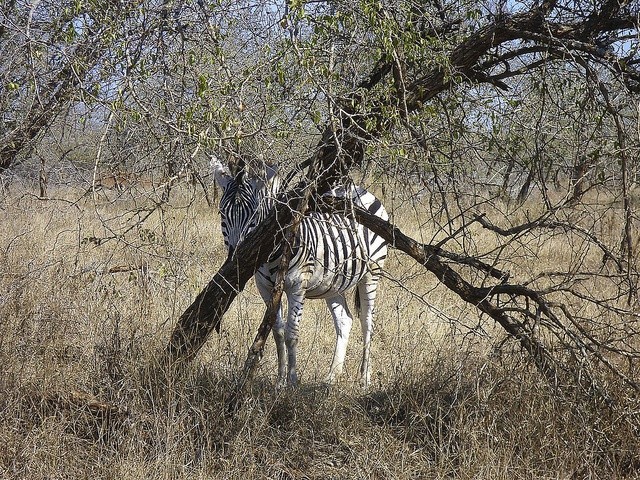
[201,154,392,395]
